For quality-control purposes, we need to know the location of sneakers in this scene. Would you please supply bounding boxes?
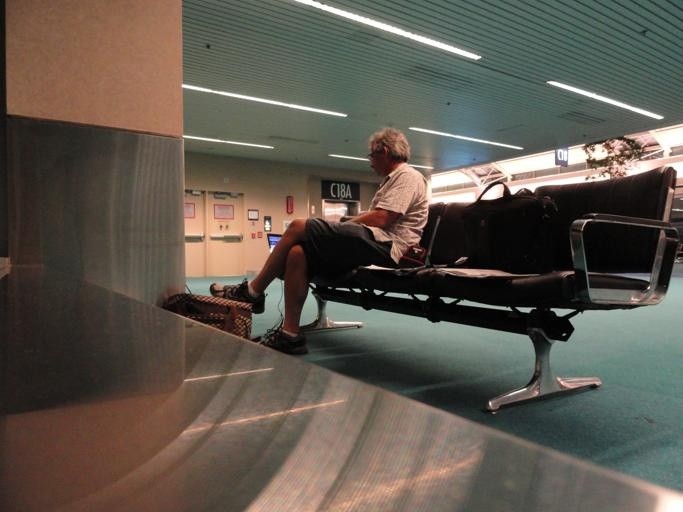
[210,281,267,315]
[255,330,310,356]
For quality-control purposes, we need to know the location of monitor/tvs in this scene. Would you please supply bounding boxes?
[267,234,282,253]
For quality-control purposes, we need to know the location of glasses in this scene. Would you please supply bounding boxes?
[367,152,383,161]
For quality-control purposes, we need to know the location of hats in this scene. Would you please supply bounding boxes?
[400,245,427,266]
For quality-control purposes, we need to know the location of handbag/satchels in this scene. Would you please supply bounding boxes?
[462,182,558,272]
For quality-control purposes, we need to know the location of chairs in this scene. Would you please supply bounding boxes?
[298,164,678,412]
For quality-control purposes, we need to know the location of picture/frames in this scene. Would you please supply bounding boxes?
[248,209,259,220]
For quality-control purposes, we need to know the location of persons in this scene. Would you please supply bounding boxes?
[210,129,429,355]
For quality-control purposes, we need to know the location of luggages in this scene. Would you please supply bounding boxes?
[164,294,254,347]
